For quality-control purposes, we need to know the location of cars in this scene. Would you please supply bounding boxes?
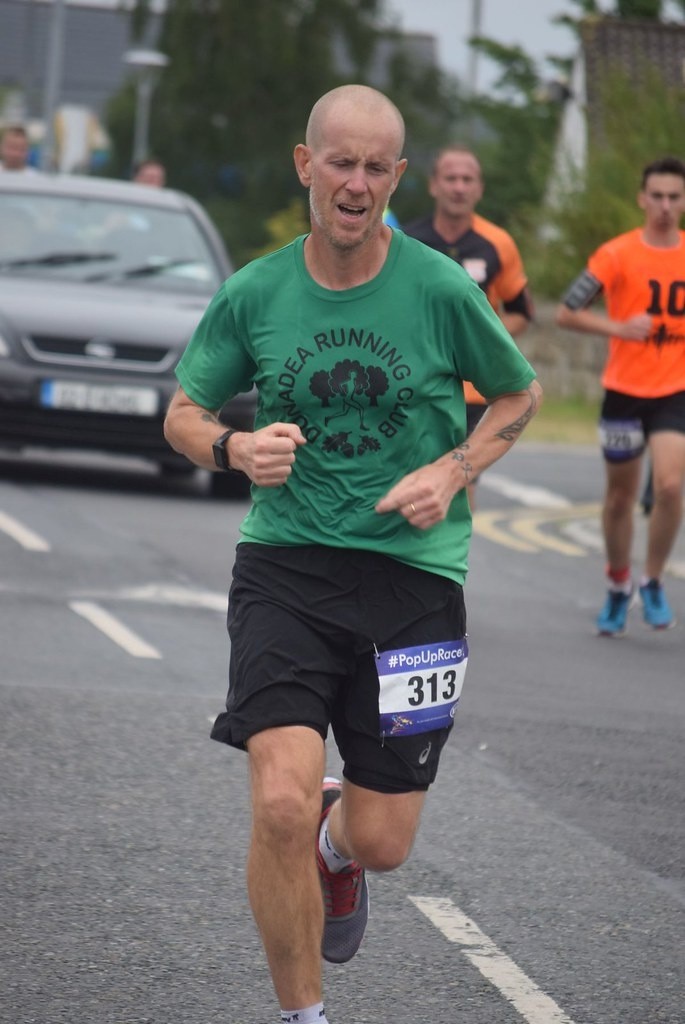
[1,179,263,494]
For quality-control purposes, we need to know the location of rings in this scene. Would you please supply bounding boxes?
[411,503,416,514]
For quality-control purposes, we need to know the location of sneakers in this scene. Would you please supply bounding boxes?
[639,578,675,632]
[316,776,370,964]
[596,582,637,638]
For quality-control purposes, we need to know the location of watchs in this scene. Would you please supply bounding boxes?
[211,429,237,472]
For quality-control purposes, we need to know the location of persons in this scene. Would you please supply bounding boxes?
[165,84,545,1024]
[555,157,685,639]
[135,159,166,187]
[0,127,38,174]
[399,147,535,440]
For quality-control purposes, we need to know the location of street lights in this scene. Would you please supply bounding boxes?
[123,49,168,174]
